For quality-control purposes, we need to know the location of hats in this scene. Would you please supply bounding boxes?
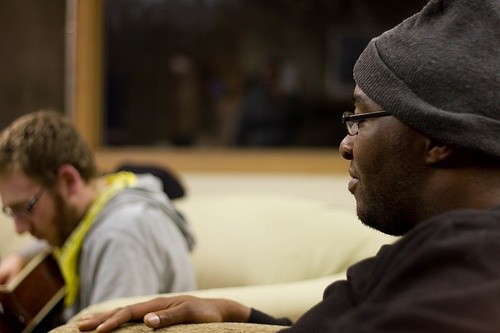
[354,0,500,162]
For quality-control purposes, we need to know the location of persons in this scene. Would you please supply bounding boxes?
[0,109,198,326]
[77,0,500,333]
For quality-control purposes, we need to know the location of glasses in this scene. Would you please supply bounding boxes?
[2,185,47,217]
[342,110,393,137]
